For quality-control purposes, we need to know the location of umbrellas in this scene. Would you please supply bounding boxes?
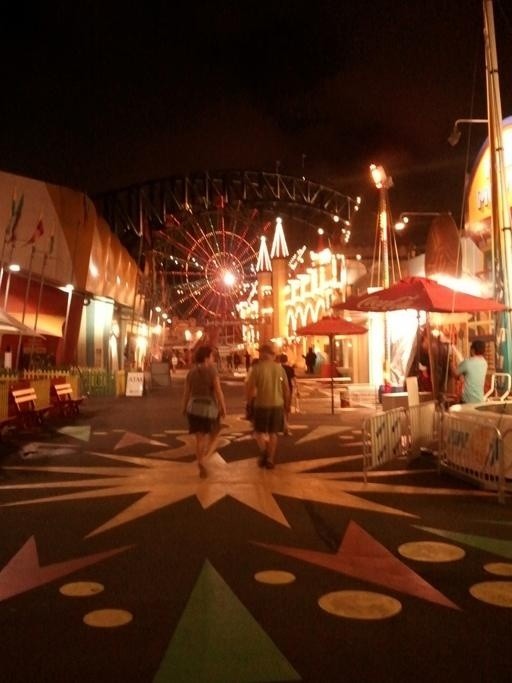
[330,276,508,400]
[296,316,369,416]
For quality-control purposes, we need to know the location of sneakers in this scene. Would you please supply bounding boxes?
[258,460,275,469]
[198,457,208,479]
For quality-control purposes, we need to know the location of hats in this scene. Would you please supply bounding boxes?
[256,345,275,355]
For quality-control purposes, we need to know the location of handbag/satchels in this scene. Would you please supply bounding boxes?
[295,394,300,415]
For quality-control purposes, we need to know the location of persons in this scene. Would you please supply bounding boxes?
[245,341,291,469]
[181,345,226,480]
[279,355,294,391]
[450,340,488,404]
[301,347,316,373]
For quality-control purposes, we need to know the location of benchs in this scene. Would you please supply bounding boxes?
[53,383,84,417]
[11,388,55,429]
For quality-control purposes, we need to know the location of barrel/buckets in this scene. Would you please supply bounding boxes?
[321,363,331,378]
